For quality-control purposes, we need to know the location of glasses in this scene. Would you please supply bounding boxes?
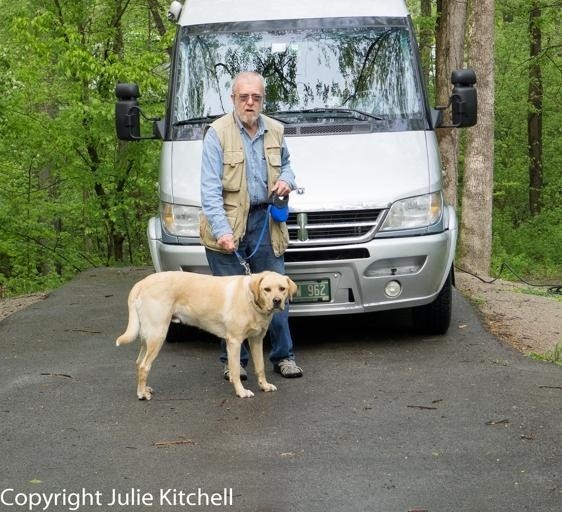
[234,93,264,102]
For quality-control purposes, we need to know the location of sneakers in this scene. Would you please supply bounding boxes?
[274,358,304,379]
[224,364,247,381]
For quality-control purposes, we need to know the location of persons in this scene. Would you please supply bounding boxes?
[200,70,303,381]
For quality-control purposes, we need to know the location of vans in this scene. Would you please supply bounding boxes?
[113,0,476,338]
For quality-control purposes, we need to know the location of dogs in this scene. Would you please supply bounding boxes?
[113,269,299,403]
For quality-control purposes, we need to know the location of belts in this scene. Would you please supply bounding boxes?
[247,203,268,214]
